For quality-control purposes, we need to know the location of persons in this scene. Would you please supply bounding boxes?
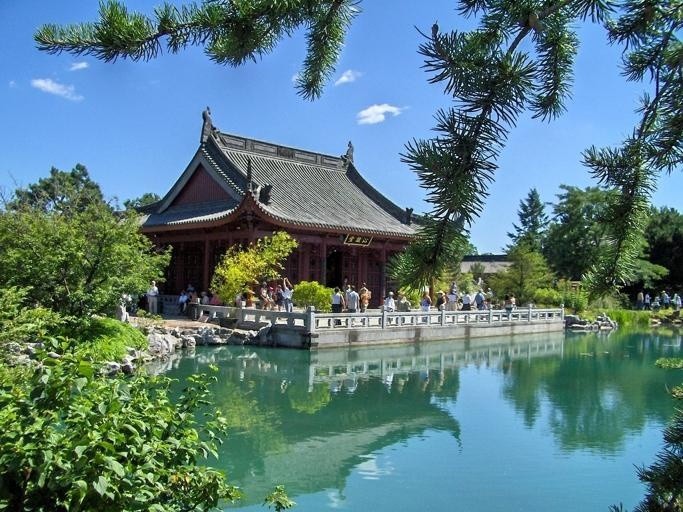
[436,282,514,322]
[422,291,432,323]
[331,282,371,324]
[382,291,411,324]
[146,280,157,315]
[178,278,294,320]
[636,289,682,311]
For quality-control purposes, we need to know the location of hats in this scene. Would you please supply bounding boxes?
[436,290,443,293]
